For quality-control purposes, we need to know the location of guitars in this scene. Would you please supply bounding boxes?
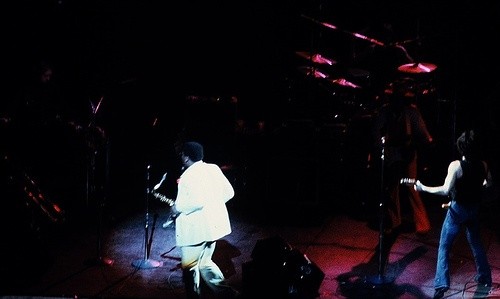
[400,177,452,208]
[146,189,175,207]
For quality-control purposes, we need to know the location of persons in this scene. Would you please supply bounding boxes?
[414,131,492,298]
[172,143,234,290]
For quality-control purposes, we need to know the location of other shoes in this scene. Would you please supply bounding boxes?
[475,276,492,288]
[432,285,449,298]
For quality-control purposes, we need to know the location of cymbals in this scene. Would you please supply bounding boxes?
[295,48,337,65]
[297,64,330,79]
[396,61,438,74]
[327,77,363,89]
